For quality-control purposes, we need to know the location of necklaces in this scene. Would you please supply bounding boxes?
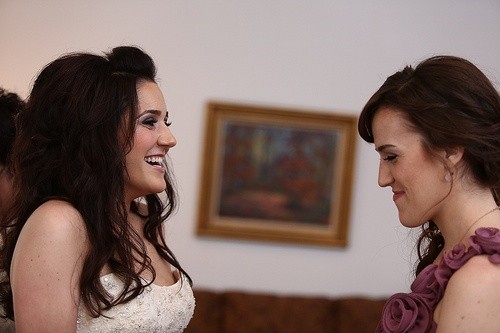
[432,205,500,264]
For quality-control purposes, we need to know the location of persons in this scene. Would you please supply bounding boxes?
[10,45,196,333]
[0,87,25,333]
[357,54,500,333]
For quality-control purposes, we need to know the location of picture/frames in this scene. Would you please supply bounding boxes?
[195,98,360,251]
